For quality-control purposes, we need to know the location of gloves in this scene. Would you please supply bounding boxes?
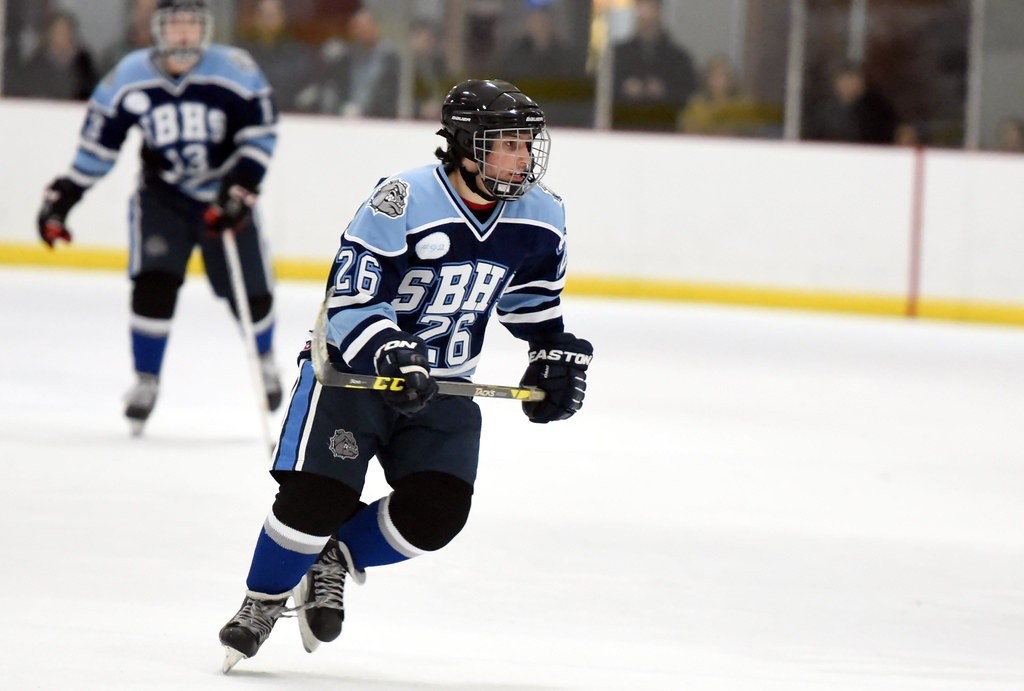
[203,161,262,234]
[39,178,86,248]
[369,331,439,418]
[519,333,593,424]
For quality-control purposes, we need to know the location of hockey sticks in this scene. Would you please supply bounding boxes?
[220,228,277,458]
[308,285,547,404]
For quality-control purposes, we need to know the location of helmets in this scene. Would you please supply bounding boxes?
[435,79,551,201]
[150,0,214,73]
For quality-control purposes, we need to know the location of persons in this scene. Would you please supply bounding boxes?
[502,8,573,75]
[95,0,158,80]
[219,79,594,674]
[37,0,285,437]
[30,13,99,102]
[680,53,782,137]
[614,0,694,131]
[238,0,311,107]
[326,11,400,120]
[369,19,456,117]
[807,66,917,146]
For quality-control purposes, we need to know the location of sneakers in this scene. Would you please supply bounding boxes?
[219,596,298,674]
[292,501,369,653]
[125,368,159,435]
[260,348,282,411]
